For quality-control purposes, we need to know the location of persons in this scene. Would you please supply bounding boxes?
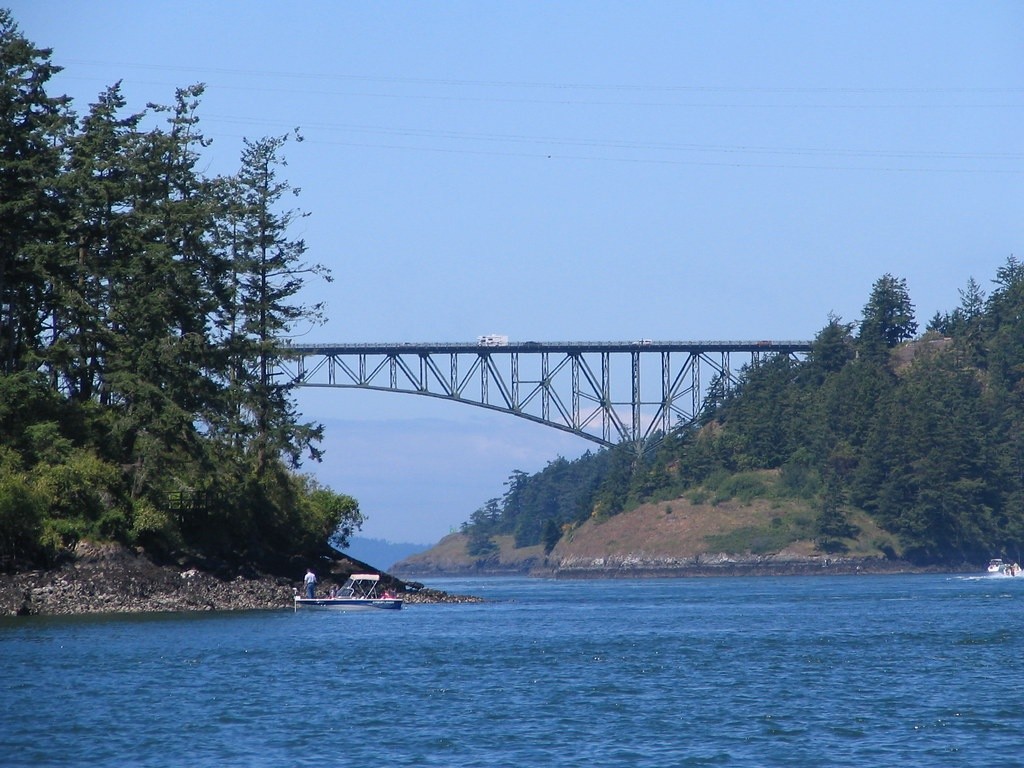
[304,568,317,599]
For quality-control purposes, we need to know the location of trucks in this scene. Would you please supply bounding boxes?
[477,334,509,349]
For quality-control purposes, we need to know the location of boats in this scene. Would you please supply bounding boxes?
[293,574,403,611]
[988,560,1022,577]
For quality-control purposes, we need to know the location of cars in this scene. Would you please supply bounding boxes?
[632,339,652,345]
